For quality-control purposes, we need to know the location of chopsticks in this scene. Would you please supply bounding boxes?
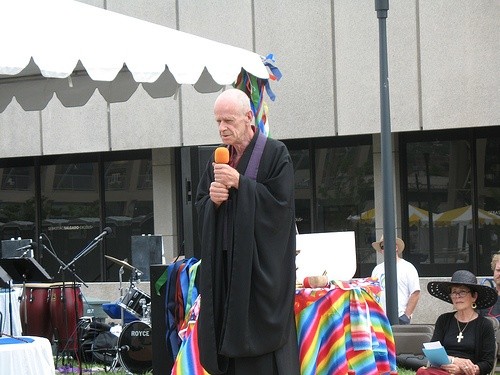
[322,271,327,276]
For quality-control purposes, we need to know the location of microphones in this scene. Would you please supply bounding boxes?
[215,147,230,165]
[118,345,130,352]
[39,235,43,258]
[94,227,112,242]
[15,242,38,251]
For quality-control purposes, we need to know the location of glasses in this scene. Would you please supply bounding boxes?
[449,291,471,299]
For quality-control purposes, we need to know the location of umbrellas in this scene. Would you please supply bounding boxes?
[348,203,436,227]
[0,0,283,115]
[435,206,500,225]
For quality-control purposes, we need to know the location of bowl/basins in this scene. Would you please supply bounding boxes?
[309,276,327,287]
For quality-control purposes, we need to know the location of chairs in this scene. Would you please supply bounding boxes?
[484,315,500,375]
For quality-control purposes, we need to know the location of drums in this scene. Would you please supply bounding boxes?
[117,320,156,375]
[19,284,53,345]
[49,280,88,353]
[116,284,150,320]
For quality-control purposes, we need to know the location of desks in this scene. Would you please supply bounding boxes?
[0,336,55,375]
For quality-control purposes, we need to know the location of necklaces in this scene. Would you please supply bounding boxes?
[457,311,474,342]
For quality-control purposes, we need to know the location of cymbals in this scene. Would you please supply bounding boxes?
[104,254,145,275]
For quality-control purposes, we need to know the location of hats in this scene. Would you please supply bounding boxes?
[427,269,498,310]
[372,234,405,256]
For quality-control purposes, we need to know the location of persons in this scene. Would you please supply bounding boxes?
[477,253,500,322]
[416,270,498,375]
[371,235,420,325]
[195,89,300,375]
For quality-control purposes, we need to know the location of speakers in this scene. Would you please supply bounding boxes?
[131,235,162,281]
[150,265,175,375]
